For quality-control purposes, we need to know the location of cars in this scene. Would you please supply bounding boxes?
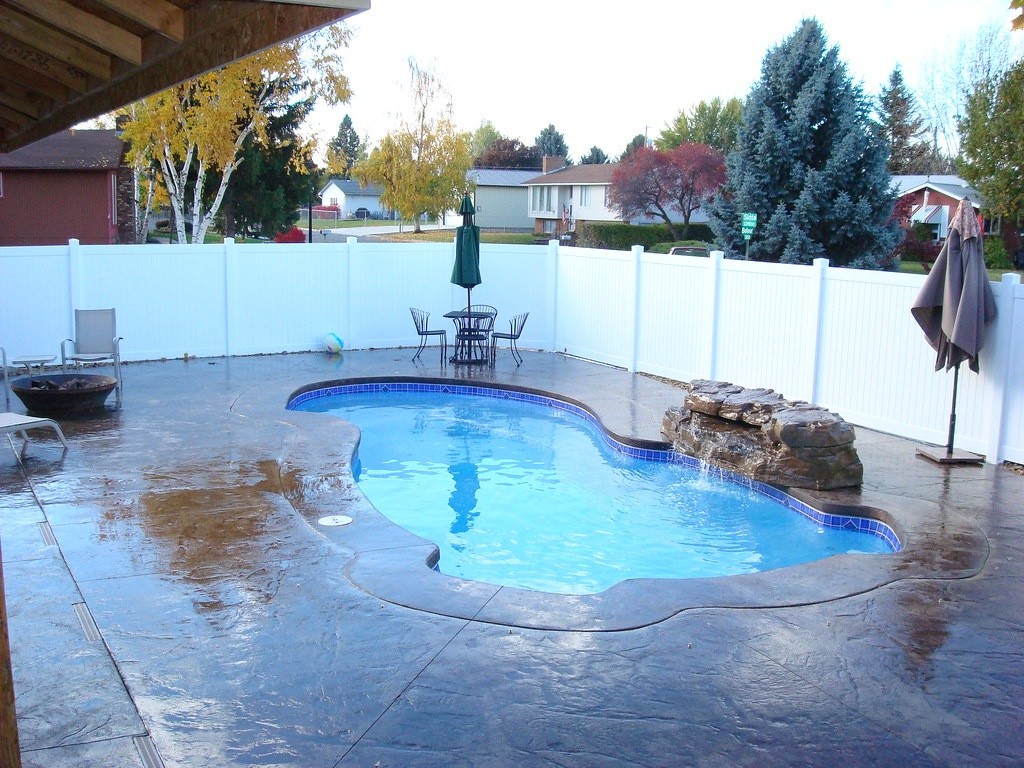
[669,247,708,257]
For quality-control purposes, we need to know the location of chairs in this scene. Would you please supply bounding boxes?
[0,412,68,450]
[0,346,9,399]
[491,312,529,366]
[409,308,446,367]
[61,307,124,388]
[460,305,498,360]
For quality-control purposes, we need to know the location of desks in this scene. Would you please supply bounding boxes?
[443,311,495,371]
[13,356,57,377]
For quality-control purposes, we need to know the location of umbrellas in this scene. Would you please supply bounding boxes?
[450,191,482,358]
[911,195,998,453]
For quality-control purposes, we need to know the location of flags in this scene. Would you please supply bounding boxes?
[562,206,566,225]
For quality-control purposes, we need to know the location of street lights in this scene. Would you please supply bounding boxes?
[302,162,314,242]
